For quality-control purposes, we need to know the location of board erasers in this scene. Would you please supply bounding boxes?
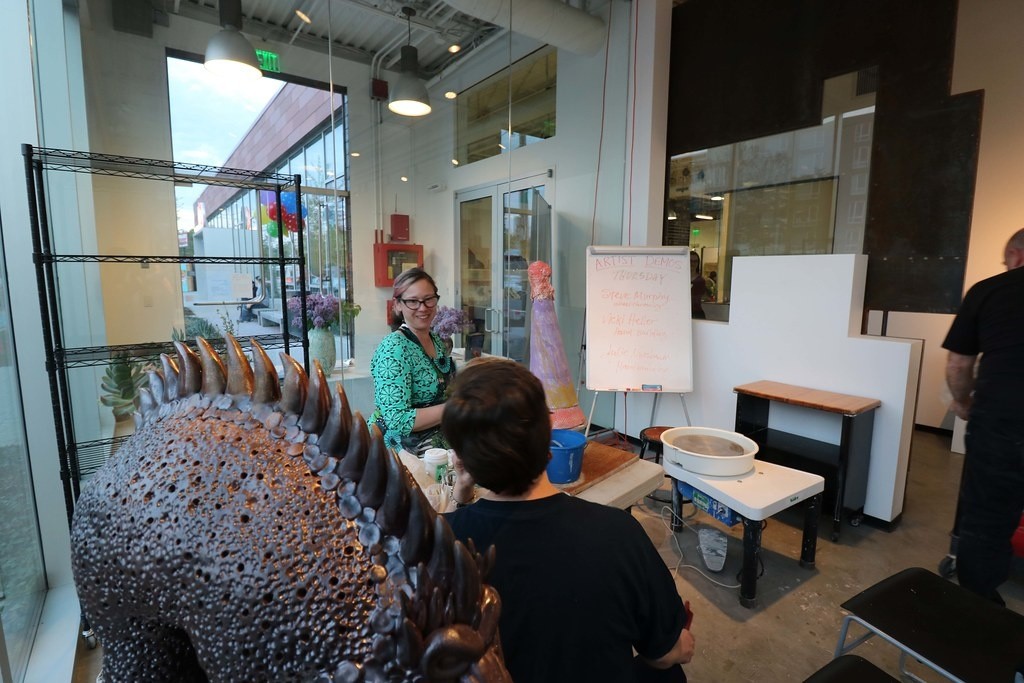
[642,384,662,391]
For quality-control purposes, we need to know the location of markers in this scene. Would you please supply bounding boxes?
[608,388,630,391]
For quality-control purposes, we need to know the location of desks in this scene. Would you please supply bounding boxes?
[552,441,665,514]
[733,379,881,544]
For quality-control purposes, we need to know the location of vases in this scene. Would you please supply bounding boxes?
[441,338,453,356]
[308,327,336,378]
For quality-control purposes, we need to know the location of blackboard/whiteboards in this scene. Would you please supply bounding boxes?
[586,246,694,393]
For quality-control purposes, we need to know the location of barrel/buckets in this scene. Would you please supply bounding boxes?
[544,429,587,484]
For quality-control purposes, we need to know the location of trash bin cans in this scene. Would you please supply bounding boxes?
[287,290,312,339]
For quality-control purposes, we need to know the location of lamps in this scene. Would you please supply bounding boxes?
[203,0,263,81]
[387,6,432,118]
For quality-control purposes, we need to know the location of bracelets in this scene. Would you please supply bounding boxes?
[449,480,478,509]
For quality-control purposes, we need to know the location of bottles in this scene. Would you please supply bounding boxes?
[424,448,448,482]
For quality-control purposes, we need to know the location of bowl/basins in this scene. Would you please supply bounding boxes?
[660,426,759,478]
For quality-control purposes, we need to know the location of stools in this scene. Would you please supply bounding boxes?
[639,426,692,505]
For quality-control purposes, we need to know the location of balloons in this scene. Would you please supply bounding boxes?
[253,186,310,238]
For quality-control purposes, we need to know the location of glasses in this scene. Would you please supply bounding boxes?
[398,294,440,310]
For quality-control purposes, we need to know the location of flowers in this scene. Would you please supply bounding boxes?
[430,305,475,340]
[287,292,362,332]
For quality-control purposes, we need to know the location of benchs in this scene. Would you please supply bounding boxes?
[252,308,273,326]
[834,566,1024,683]
[663,455,825,610]
[260,310,283,333]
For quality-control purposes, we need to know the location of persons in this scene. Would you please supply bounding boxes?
[240,276,269,321]
[438,361,696,683]
[365,268,456,460]
[941,227,1024,588]
[689,251,717,320]
